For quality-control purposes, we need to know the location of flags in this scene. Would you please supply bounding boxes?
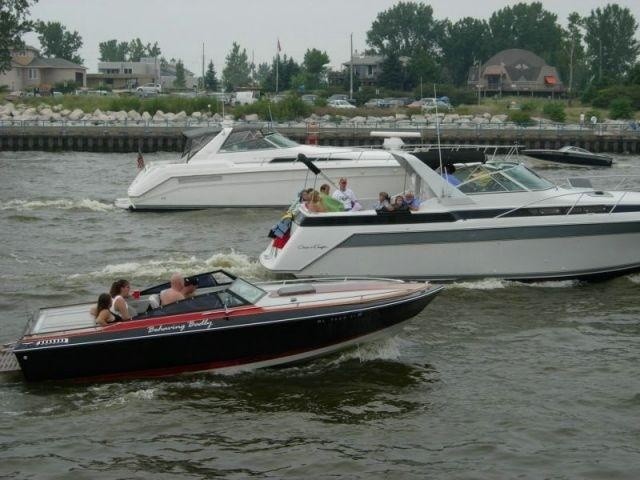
[137,151,145,169]
[278,40,281,51]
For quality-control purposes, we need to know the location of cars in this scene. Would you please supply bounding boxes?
[179,88,453,114]
[6,82,164,102]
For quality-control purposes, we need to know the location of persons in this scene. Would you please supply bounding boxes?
[90,293,123,326]
[440,163,465,192]
[579,111,586,130]
[160,272,195,307]
[109,279,134,320]
[591,114,598,129]
[300,177,421,212]
[305,134,320,145]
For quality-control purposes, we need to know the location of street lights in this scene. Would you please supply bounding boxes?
[473,59,481,108]
[563,35,574,92]
[596,37,604,83]
[202,41,206,88]
[350,32,357,99]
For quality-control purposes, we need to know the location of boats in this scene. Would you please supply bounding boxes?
[258,147,639,292]
[109,112,524,217]
[0,265,446,395]
[516,140,616,170]
